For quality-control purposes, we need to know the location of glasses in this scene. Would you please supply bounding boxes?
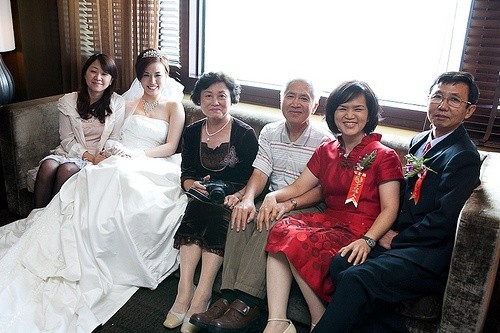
[428,94,471,108]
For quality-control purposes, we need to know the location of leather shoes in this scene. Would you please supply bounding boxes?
[206,299,263,333]
[189,297,232,329]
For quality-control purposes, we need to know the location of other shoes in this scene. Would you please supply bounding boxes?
[268,318,296,333]
[181,297,212,333]
[163,285,196,329]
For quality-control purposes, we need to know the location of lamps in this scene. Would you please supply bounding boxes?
[0,0,16,104]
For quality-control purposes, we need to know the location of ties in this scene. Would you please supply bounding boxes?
[422,142,431,156]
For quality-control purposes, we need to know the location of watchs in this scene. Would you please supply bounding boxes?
[360,236,376,250]
[289,198,297,211]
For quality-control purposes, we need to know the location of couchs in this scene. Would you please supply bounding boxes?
[1,93,500,333]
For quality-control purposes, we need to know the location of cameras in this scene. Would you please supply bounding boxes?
[200,180,228,202]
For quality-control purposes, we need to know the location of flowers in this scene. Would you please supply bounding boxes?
[402,153,439,179]
[338,149,377,175]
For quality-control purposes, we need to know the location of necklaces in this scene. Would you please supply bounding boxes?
[206,117,231,143]
[142,96,162,117]
[341,136,362,158]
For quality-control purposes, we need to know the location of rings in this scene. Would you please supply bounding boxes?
[232,204,236,208]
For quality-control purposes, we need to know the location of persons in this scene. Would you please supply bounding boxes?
[189,78,338,333]
[255,79,405,333]
[33,53,128,213]
[163,71,271,333]
[312,71,482,333]
[15,48,188,290]
